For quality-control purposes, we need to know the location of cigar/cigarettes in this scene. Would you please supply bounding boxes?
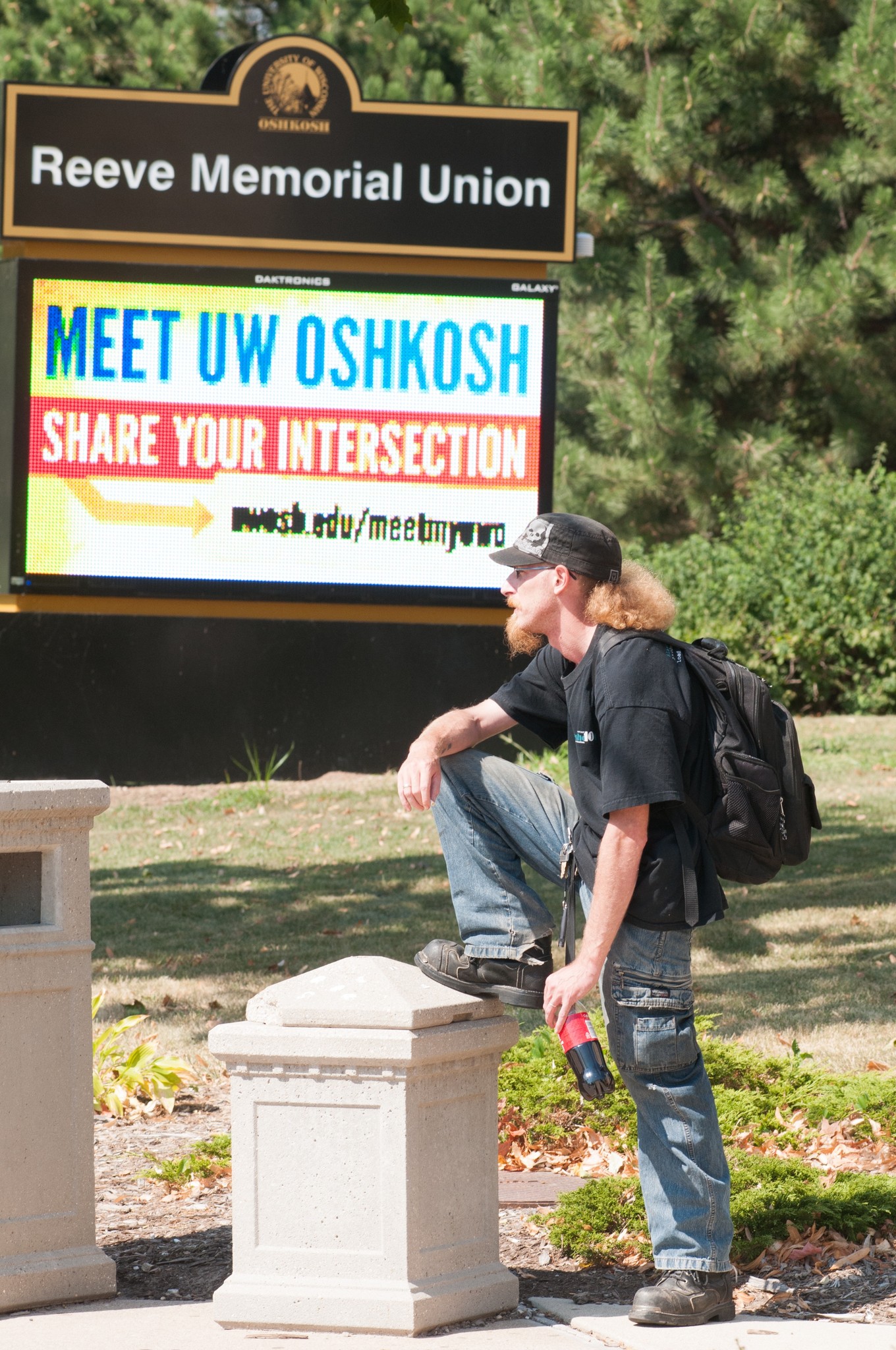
[430,800,435,806]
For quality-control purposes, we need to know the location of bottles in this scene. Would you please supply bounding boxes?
[552,999,615,1102]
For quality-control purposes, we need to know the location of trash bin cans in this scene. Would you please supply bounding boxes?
[0,780,119,1314]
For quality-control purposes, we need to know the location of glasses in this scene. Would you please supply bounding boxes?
[508,566,576,581]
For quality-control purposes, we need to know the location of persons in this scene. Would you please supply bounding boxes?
[396,512,824,1327]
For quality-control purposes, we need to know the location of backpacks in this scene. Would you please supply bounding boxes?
[570,634,824,884]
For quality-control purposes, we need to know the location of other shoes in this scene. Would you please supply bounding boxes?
[629,1266,736,1325]
[415,936,553,1010]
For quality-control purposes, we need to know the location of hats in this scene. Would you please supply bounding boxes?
[488,512,623,585]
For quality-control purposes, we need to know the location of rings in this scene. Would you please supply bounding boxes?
[402,783,412,788]
[549,1002,557,1007]
